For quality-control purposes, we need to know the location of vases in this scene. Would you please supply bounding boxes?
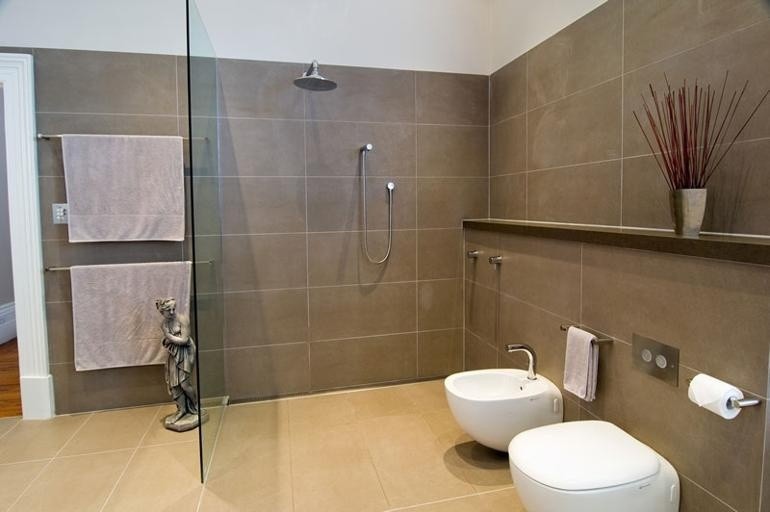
[665,178,709,240]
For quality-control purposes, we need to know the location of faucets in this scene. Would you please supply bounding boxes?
[505,342,537,380]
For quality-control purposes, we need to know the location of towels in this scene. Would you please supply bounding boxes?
[557,324,601,403]
[66,260,193,374]
[59,131,186,246]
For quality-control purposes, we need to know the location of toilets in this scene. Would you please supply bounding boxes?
[505,418,683,512]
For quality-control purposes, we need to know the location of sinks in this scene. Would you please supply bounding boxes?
[442,366,566,455]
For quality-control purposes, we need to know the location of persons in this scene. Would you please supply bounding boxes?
[154,297,210,424]
